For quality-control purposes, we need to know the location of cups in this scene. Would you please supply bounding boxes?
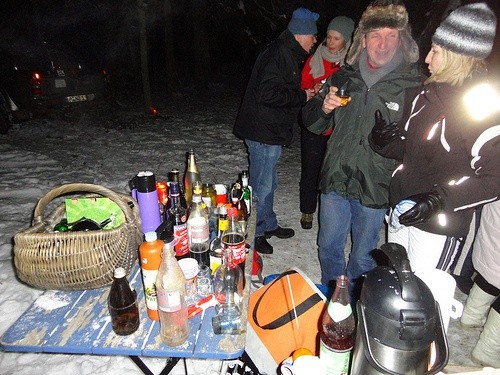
[337,89,350,106]
[212,315,245,335]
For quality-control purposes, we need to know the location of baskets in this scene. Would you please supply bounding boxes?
[13,183,143,290]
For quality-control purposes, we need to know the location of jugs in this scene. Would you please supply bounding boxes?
[348,242,450,375]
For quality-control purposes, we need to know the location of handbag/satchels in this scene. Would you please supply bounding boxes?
[243,265,330,375]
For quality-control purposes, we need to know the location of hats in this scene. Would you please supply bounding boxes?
[432,2,497,60]
[288,8,319,35]
[327,17,355,41]
[345,0,420,64]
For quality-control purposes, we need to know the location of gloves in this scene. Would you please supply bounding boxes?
[392,191,444,227]
[372,109,401,148]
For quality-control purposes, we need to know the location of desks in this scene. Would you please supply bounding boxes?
[0,199,258,375]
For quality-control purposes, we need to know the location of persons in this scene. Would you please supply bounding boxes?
[232,8,317,254]
[301,0,500,368]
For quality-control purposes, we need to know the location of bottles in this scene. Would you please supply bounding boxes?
[202,183,217,218]
[241,170,251,217]
[319,275,355,375]
[231,180,248,239]
[107,267,140,336]
[212,249,244,321]
[184,147,201,203]
[168,192,190,260]
[155,245,190,346]
[187,196,210,270]
[163,168,187,222]
[192,181,208,219]
[132,171,160,233]
[209,208,230,293]
[139,232,165,320]
[220,208,246,289]
[166,182,186,221]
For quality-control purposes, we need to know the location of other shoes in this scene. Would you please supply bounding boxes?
[264,226,294,239]
[468,353,496,369]
[300,212,313,229]
[460,321,483,328]
[253,237,273,254]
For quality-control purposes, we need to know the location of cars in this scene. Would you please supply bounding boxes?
[0,43,108,135]
